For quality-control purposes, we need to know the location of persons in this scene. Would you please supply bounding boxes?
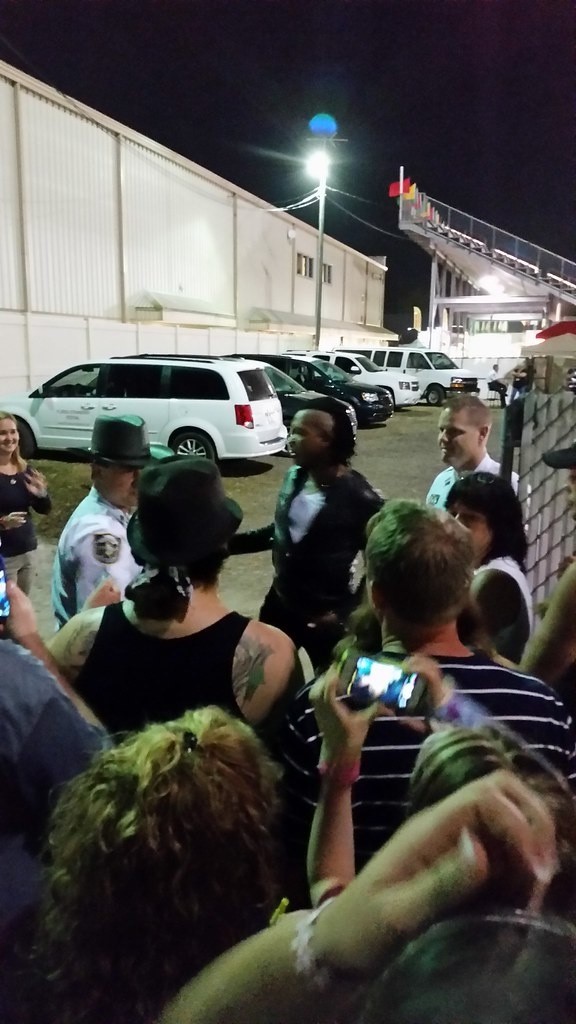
[0,396,576,1024]
[487,357,535,409]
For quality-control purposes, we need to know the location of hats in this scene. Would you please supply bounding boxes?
[66,414,174,470]
[126,456,243,564]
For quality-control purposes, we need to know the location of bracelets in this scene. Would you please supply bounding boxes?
[289,897,333,1007]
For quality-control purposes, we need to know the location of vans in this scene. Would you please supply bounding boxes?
[334,347,481,408]
[0,357,289,468]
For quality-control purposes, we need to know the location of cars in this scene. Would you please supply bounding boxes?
[282,349,427,410]
[252,360,358,451]
[219,354,395,427]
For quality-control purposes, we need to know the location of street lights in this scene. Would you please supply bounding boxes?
[305,133,334,354]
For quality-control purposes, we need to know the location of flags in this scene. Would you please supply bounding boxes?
[389,178,442,225]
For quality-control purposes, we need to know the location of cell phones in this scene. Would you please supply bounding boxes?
[0,555,10,624]
[335,649,427,717]
